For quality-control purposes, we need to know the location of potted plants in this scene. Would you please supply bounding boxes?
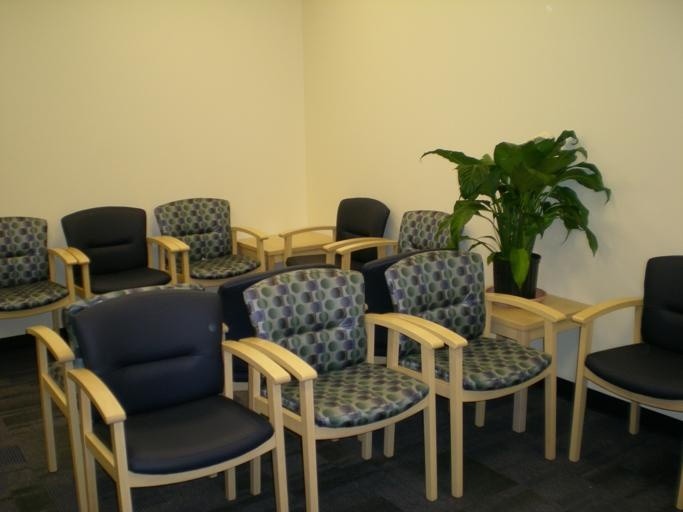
[418,128,611,300]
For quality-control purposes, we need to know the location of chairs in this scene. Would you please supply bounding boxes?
[568,255,683,512]
[385,250,566,498]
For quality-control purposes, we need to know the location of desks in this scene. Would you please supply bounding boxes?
[475,289,593,433]
